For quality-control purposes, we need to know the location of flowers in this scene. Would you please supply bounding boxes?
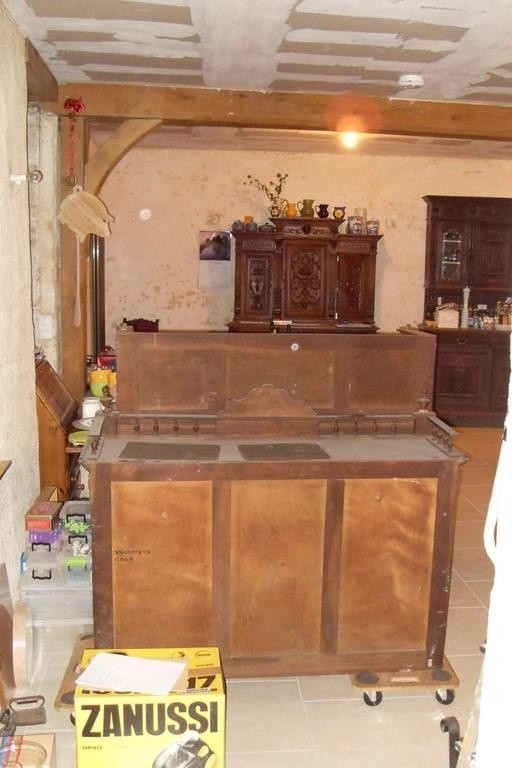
[242,172,291,204]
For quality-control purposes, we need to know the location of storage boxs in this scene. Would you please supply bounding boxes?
[20,567,65,589]
[58,500,92,531]
[25,501,64,533]
[433,309,459,328]
[60,528,92,557]
[28,519,64,545]
[26,542,63,565]
[62,556,92,583]
[74,646,226,768]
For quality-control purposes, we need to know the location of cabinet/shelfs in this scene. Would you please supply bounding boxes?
[420,194,511,429]
[230,218,384,334]
[35,357,83,501]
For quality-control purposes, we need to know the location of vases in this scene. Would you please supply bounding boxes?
[266,198,288,219]
[283,202,298,219]
[333,206,347,220]
[297,199,316,217]
[315,203,330,218]
